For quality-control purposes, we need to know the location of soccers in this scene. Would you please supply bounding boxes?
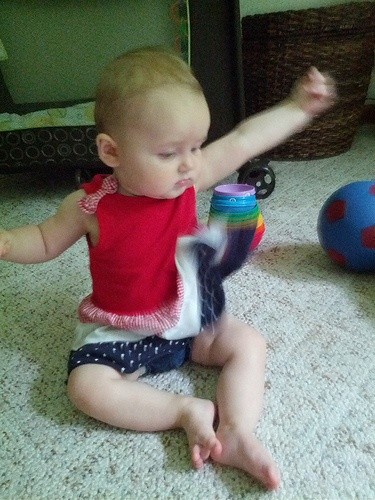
[317,180,375,274]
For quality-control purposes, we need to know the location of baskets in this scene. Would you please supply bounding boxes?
[241,1,375,162]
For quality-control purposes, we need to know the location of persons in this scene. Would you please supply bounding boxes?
[0,43,338,491]
